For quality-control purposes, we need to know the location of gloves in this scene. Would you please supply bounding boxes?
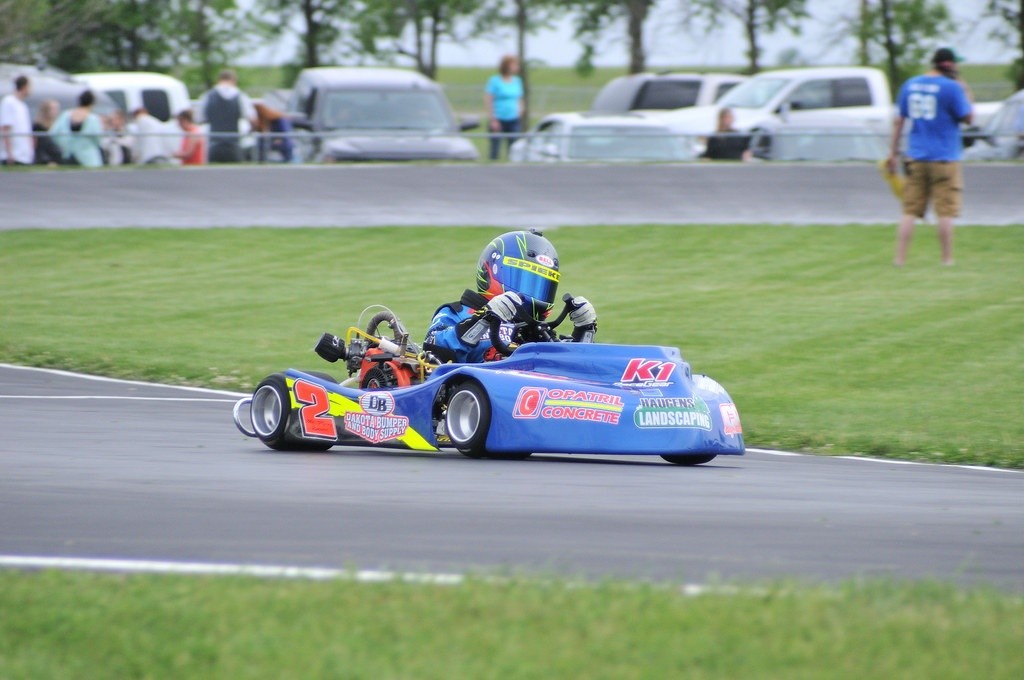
[455,292,522,348]
[562,297,597,343]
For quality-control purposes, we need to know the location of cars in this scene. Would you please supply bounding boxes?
[508,112,694,169]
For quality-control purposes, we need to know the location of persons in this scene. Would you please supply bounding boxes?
[421,229,598,366]
[701,110,753,161]
[886,47,972,265]
[487,57,527,160]
[0,68,298,169]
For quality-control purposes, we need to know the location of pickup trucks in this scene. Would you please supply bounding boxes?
[660,68,1001,167]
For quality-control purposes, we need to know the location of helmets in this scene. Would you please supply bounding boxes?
[475,228,560,321]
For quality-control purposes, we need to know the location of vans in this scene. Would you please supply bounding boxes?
[71,70,190,168]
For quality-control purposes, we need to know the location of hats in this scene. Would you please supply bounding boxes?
[932,48,966,63]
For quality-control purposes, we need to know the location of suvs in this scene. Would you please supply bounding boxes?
[0,63,121,166]
[281,62,481,164]
[597,71,742,113]
